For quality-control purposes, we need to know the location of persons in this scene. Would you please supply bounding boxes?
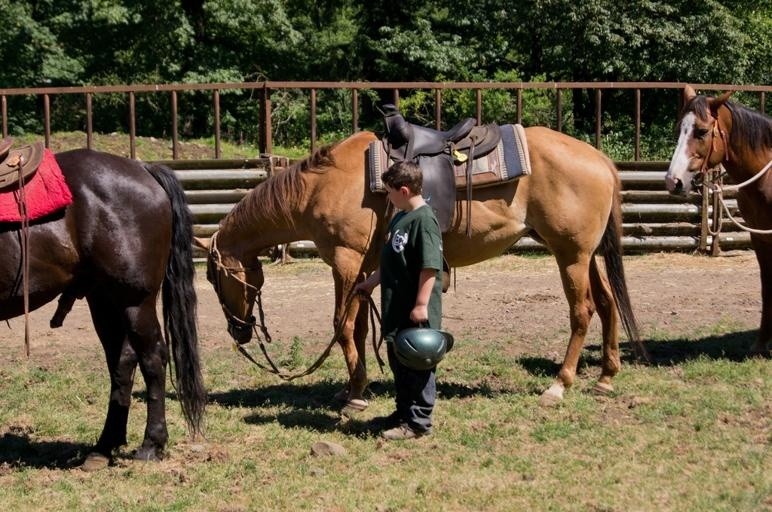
[351,162,445,440]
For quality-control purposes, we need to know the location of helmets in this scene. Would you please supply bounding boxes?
[394,328,454,371]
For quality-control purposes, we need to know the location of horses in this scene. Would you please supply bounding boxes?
[0,147,207,472]
[664,83,772,359]
[190,125,649,412]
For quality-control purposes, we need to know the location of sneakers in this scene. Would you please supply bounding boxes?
[369,416,401,432]
[384,424,433,439]
